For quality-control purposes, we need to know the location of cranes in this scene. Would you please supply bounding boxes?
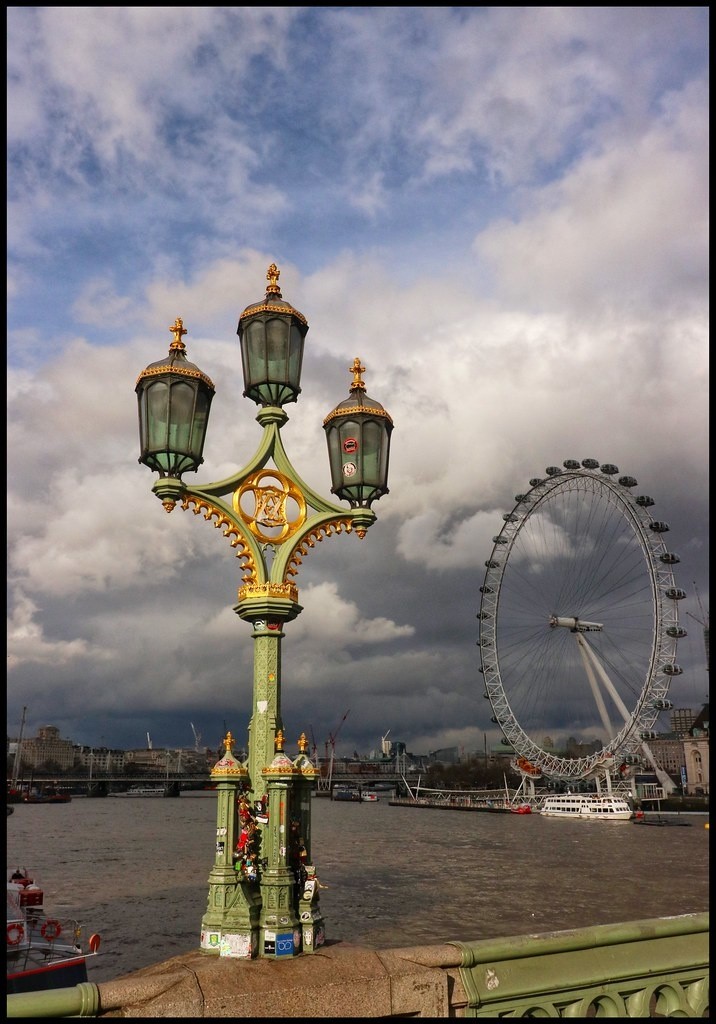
[310,709,351,769]
[190,720,202,753]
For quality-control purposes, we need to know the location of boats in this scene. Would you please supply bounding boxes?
[6,866,101,996]
[163,782,180,798]
[540,795,633,820]
[332,787,378,803]
[7,706,73,804]
[511,803,531,815]
[87,781,109,797]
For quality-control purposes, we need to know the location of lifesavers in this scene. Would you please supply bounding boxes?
[7,924,24,945]
[41,920,61,940]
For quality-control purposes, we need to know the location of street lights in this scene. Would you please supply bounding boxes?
[134,260,395,958]
